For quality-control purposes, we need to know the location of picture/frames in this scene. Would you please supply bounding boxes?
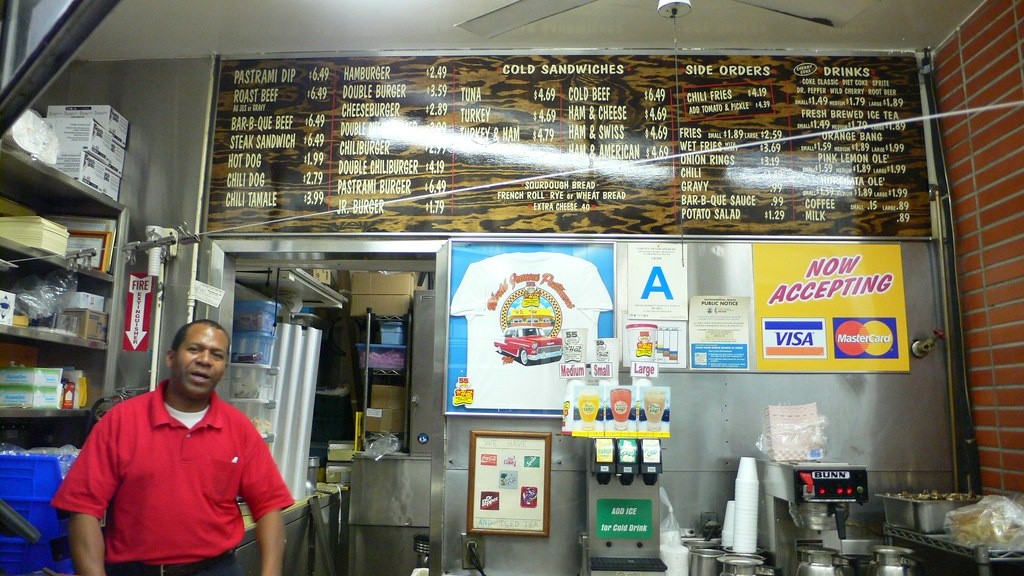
[467,432,550,536]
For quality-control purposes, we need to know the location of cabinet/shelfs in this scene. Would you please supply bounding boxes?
[1,143,132,420]
[229,362,279,443]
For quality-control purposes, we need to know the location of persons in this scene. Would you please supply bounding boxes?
[47,318,296,576]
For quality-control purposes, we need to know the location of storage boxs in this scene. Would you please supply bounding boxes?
[0,104,132,408]
[232,300,282,364]
[345,272,420,439]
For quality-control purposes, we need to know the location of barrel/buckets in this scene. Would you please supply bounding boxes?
[683,538,919,576]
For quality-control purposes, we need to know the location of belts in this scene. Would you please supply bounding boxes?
[106,550,234,576]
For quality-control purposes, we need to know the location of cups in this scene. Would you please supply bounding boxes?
[722,457,761,553]
[609,388,633,430]
[579,392,602,431]
[641,391,665,429]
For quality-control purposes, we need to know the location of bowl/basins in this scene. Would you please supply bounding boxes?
[874,493,982,534]
